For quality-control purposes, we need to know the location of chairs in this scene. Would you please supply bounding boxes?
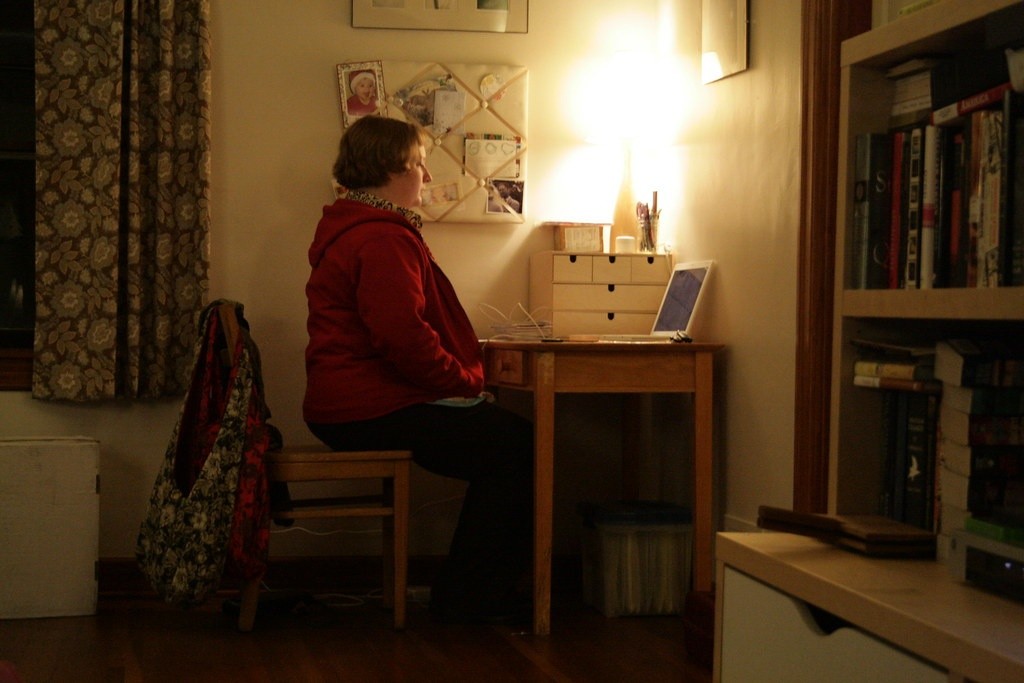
[210,299,414,631]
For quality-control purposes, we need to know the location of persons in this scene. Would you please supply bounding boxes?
[302,115,538,628]
[497,180,521,212]
[409,97,426,121]
[347,70,381,116]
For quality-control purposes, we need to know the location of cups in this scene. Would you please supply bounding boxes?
[616,237,635,252]
[638,220,658,254]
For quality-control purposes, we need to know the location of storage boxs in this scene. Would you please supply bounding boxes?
[577,500,695,620]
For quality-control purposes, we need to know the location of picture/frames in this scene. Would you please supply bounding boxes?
[352,0,529,34]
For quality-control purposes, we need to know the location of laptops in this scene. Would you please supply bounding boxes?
[570,258,718,342]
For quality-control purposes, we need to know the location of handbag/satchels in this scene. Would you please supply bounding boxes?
[136,306,253,610]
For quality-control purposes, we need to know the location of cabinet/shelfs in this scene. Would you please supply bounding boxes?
[714,0,1024,683]
[528,249,674,338]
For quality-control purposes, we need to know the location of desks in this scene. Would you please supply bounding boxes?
[478,335,724,637]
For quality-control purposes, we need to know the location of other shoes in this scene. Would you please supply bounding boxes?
[441,597,528,626]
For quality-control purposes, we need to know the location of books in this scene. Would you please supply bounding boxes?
[850,36,1024,287]
[850,337,1024,606]
